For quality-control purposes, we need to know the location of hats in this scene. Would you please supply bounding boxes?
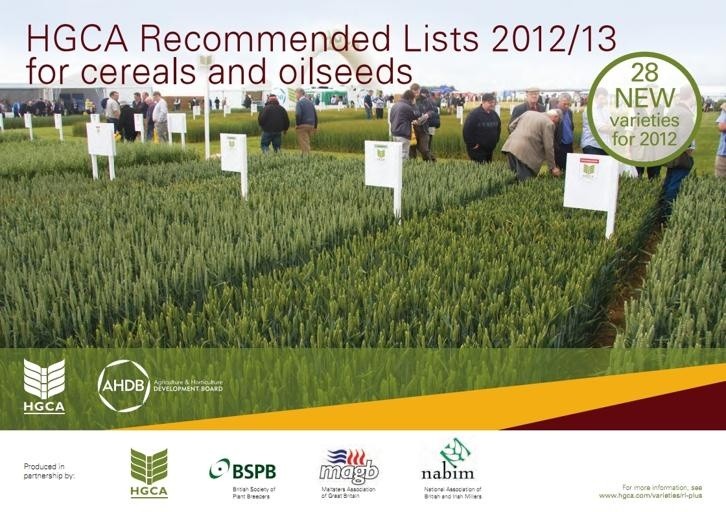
[526,87,540,92]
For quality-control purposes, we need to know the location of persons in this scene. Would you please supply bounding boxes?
[502,86,608,186]
[173,95,181,110]
[659,133,696,217]
[257,93,290,153]
[371,89,386,119]
[294,87,318,157]
[0,90,170,146]
[364,89,375,121]
[462,93,502,163]
[190,97,199,109]
[241,95,252,109]
[330,95,344,107]
[636,166,661,180]
[714,106,726,179]
[387,82,468,162]
[701,95,714,114]
[214,97,220,110]
